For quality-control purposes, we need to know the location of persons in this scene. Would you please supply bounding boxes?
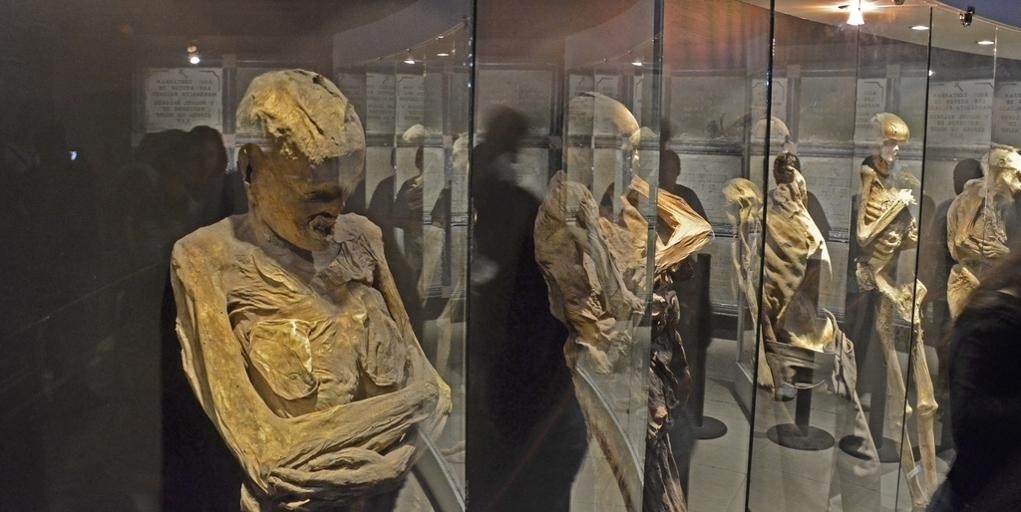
[532,92,714,512]
[168,69,453,512]
